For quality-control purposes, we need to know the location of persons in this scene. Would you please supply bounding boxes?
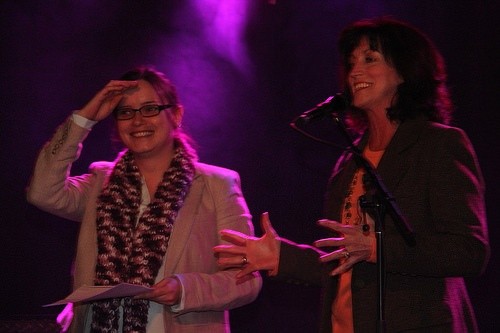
[211,16,490,333]
[28,67,264,333]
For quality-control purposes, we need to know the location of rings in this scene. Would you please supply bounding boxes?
[241,256,247,265]
[342,247,351,260]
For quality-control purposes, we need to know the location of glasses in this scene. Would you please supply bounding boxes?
[113,103,173,120]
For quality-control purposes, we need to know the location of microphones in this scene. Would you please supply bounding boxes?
[294,93,347,128]
